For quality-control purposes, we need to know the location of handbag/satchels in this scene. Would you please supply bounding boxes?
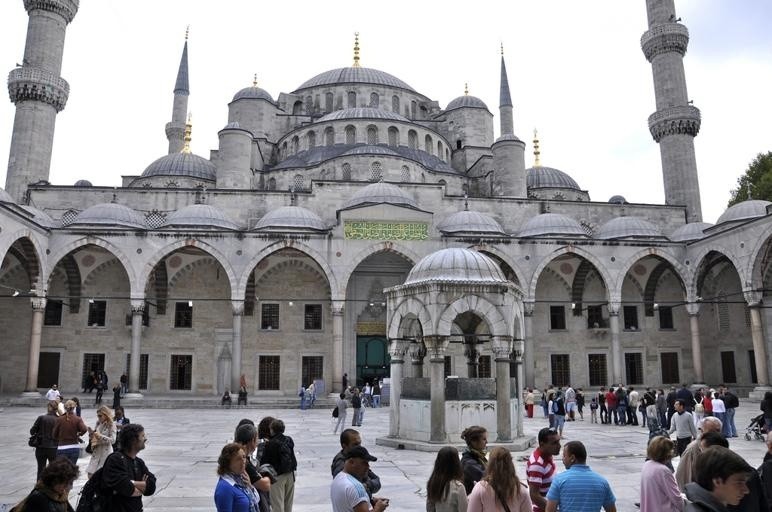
[29,435,41,446]
[75,452,128,512]
[86,439,96,453]
[333,407,338,417]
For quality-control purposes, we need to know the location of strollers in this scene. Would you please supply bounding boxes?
[744,414,768,443]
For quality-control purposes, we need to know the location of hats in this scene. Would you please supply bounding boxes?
[52,385,57,390]
[346,447,377,461]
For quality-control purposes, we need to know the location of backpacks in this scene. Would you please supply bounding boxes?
[694,398,704,416]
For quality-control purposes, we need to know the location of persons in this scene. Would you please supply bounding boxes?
[425,382,772,511]
[298,372,381,433]
[220,372,249,405]
[9,367,157,512]
[214,417,389,512]
